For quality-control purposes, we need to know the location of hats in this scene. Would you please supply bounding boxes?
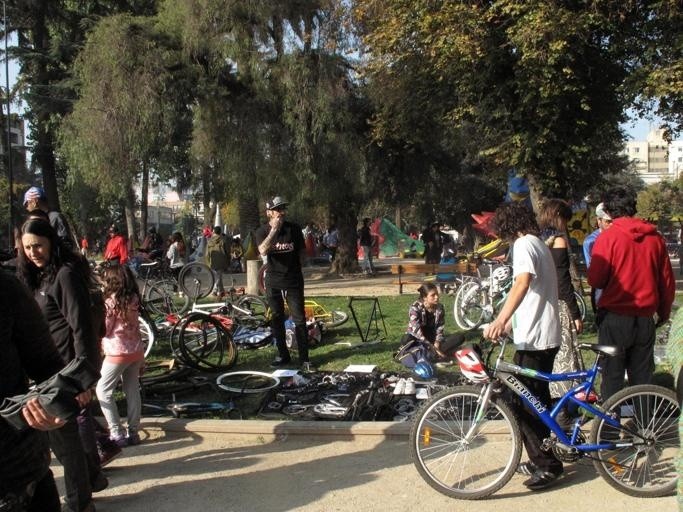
[266,196,289,210]
[22,186,45,205]
[595,202,612,221]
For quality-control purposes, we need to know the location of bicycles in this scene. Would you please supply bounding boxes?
[408,334,682,501]
[451,259,587,335]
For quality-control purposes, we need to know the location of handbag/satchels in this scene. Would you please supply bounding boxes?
[394,340,436,369]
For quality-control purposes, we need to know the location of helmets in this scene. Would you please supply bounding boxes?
[453,343,492,384]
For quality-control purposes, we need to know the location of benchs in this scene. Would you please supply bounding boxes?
[389,262,478,295]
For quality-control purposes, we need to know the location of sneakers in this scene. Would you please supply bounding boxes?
[523,467,564,490]
[60,499,97,512]
[300,360,318,373]
[97,443,122,468]
[270,356,291,369]
[110,431,140,446]
[63,476,109,502]
[515,460,538,475]
[172,292,226,299]
